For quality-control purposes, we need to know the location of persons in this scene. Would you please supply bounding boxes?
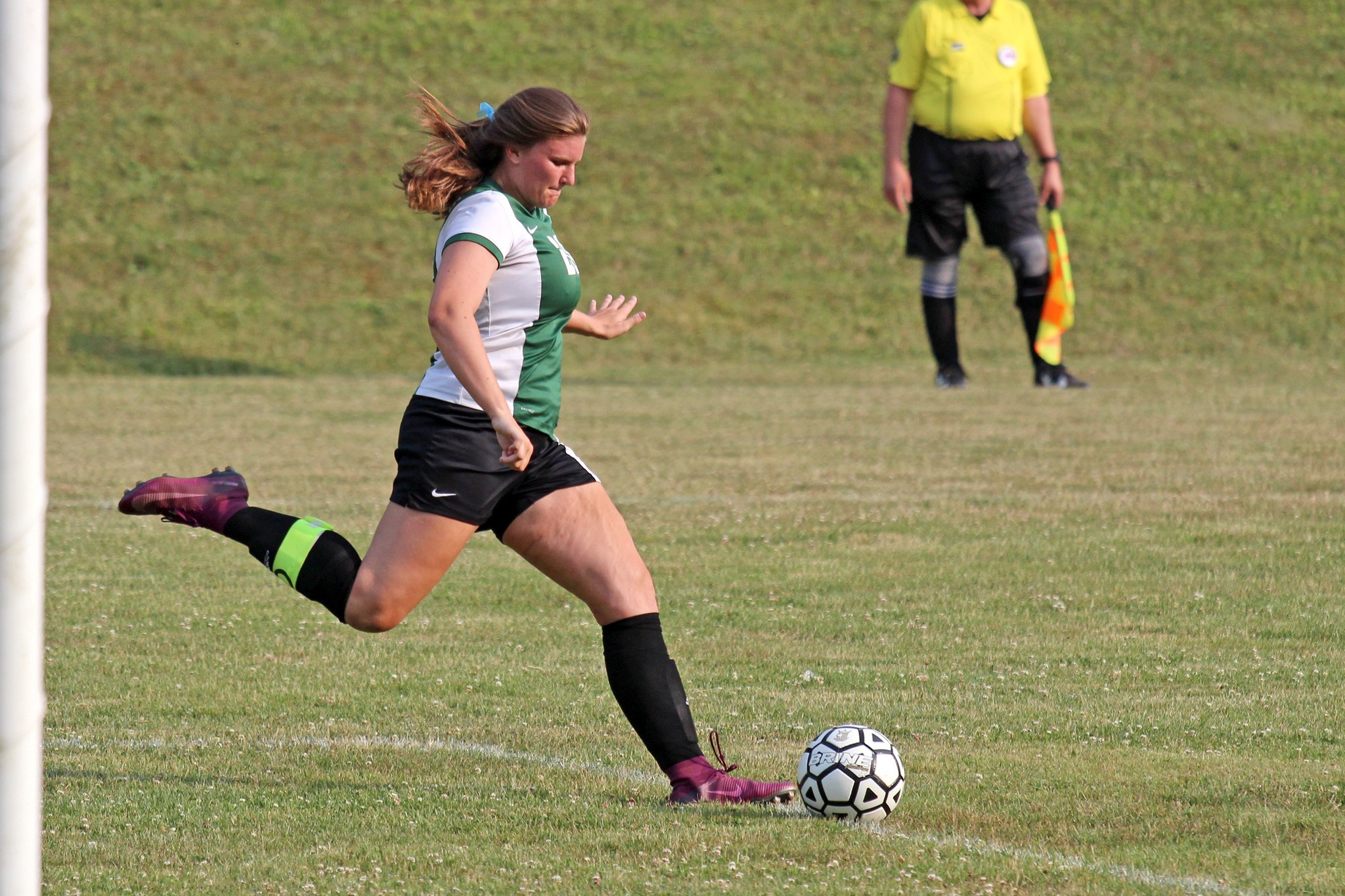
[879,0,1086,390]
[118,87,798,808]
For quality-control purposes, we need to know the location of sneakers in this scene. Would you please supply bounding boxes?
[668,730,797,805]
[118,466,250,527]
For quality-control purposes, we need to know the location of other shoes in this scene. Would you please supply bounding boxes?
[1034,365,1090,389]
[933,362,966,387]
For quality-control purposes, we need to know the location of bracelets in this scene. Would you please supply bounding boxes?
[1037,152,1062,166]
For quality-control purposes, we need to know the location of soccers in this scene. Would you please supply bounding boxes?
[796,724,906,824]
